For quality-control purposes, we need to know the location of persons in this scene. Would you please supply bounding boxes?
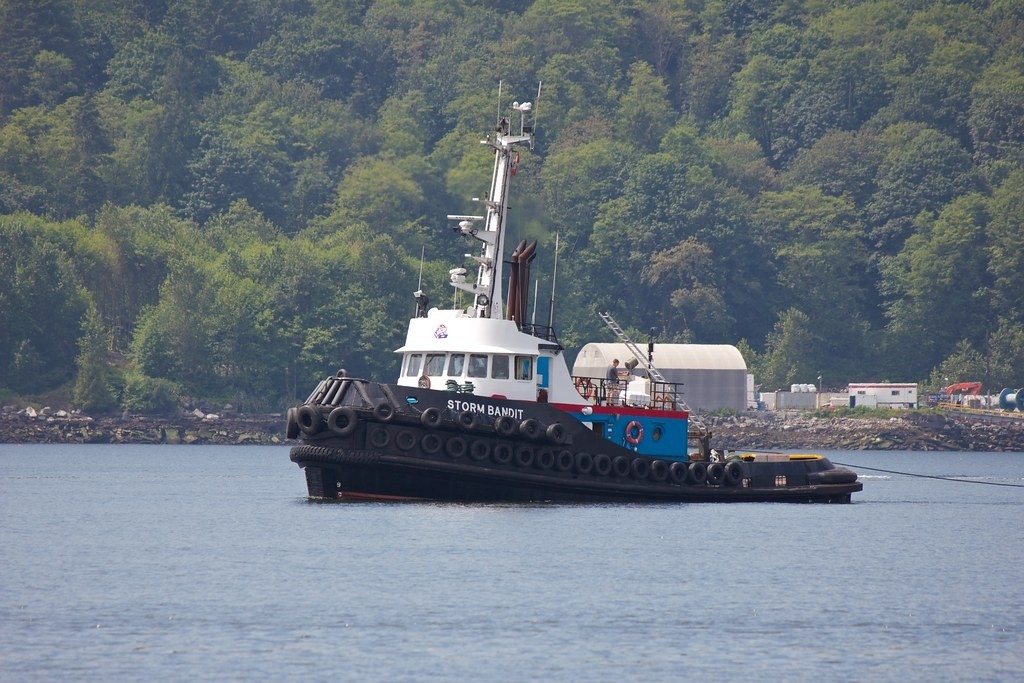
[606,359,619,406]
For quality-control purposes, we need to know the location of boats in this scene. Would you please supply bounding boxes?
[284,76,865,506]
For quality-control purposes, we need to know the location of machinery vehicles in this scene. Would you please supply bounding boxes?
[927,383,982,405]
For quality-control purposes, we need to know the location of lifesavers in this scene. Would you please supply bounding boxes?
[625,419,643,443]
[574,377,593,401]
[575,452,744,486]
[367,401,575,471]
[283,402,359,441]
